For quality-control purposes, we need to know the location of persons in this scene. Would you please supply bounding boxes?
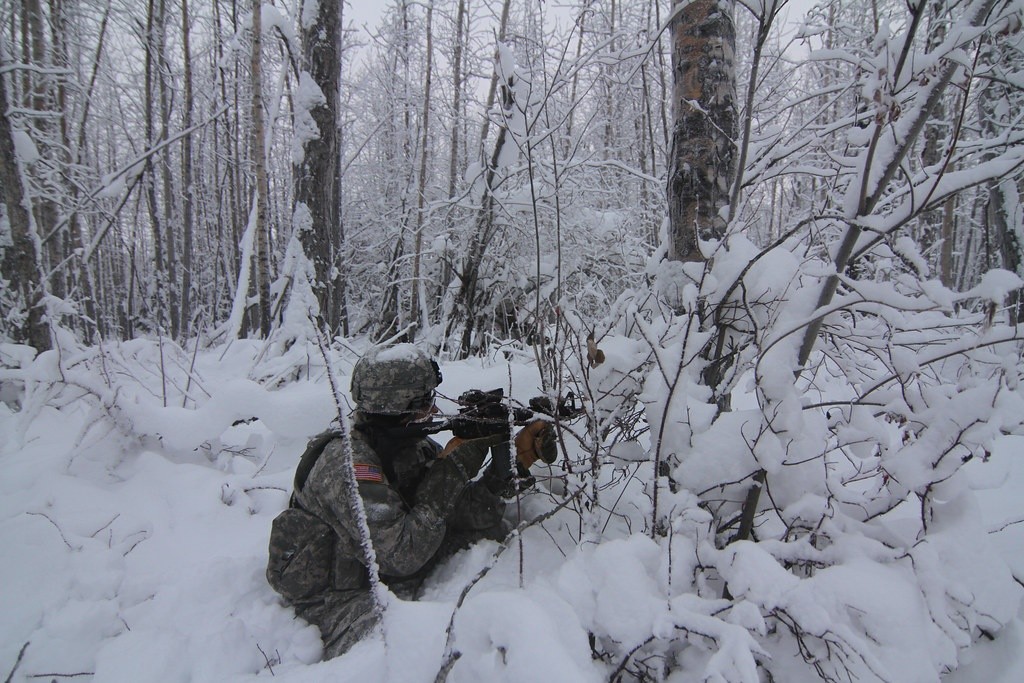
[267,343,557,662]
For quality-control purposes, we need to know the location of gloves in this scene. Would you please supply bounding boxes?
[513,419,557,470]
[436,433,505,480]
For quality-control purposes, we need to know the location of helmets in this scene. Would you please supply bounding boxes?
[350,343,443,416]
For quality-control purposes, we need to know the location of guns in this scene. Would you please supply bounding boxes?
[404,387,584,500]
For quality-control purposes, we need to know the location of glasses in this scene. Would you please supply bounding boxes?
[409,392,437,413]
